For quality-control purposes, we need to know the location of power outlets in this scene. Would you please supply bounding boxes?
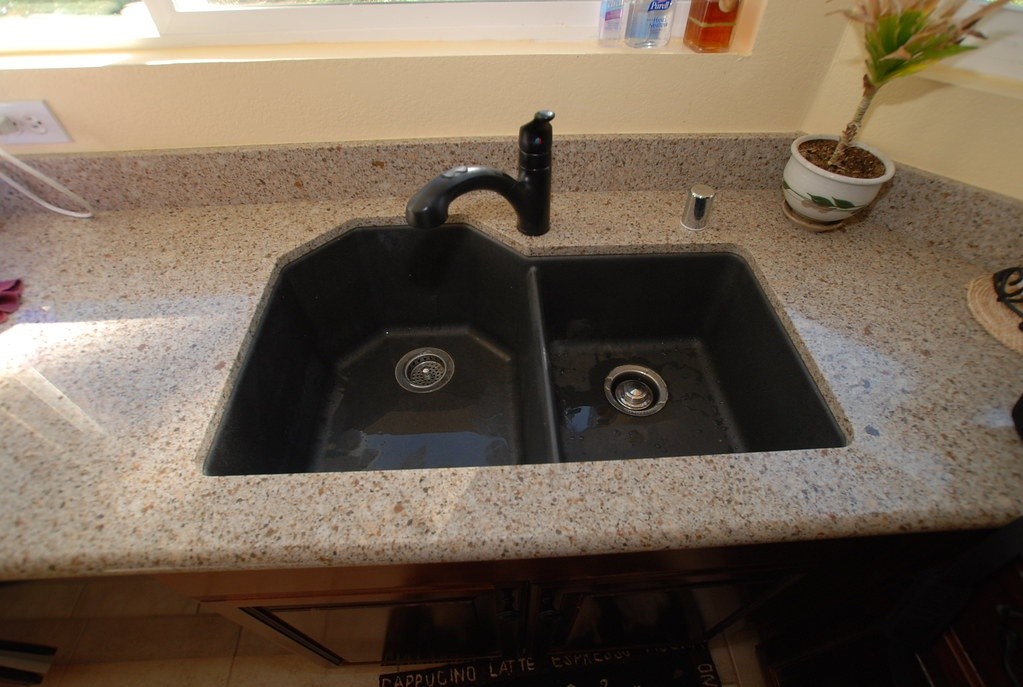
[0,100,73,148]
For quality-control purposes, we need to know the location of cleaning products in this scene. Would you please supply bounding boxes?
[598,0,625,47]
[623,0,677,49]
[682,0,744,54]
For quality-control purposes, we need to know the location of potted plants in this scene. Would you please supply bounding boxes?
[778,1,1023,234]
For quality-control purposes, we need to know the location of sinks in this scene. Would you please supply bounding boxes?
[196,215,556,477]
[532,242,857,472]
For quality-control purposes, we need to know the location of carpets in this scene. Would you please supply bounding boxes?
[379,587,722,686]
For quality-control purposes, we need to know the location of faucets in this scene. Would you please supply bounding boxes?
[405,110,556,238]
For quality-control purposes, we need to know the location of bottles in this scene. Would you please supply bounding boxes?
[598,0,625,47]
[682,0,742,54]
[624,0,677,48]
